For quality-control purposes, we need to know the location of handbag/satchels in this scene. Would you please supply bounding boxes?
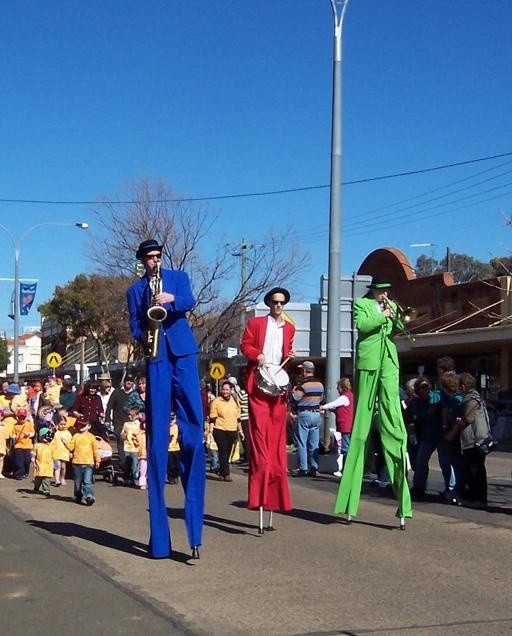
[228,430,243,464]
[473,431,499,455]
[94,435,113,463]
[9,445,16,456]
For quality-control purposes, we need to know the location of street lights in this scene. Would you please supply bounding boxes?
[407,239,452,271]
[1,219,91,388]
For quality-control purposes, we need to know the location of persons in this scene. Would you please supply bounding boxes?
[332,276,414,520]
[238,287,296,513]
[126,237,204,559]
[200,357,511,507]
[1,361,180,506]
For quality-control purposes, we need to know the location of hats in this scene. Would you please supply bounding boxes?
[98,373,113,380]
[64,374,75,387]
[39,427,49,437]
[124,373,135,382]
[76,415,89,425]
[136,239,163,260]
[7,383,21,395]
[297,360,315,371]
[365,276,393,289]
[17,409,27,417]
[263,287,290,307]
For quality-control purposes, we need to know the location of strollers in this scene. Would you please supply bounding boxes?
[81,414,136,486]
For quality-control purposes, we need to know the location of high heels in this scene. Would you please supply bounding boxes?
[132,480,140,489]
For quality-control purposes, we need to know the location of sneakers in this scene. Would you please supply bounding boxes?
[124,480,129,485]
[38,489,51,496]
[370,479,488,510]
[333,471,342,477]
[85,497,95,506]
[290,469,307,477]
[55,479,66,485]
[209,467,232,482]
[76,493,87,505]
[307,466,316,477]
[140,485,146,489]
[34,488,40,494]
[169,477,178,485]
[363,472,372,479]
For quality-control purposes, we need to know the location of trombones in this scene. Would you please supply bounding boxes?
[148,260,168,358]
[381,294,418,345]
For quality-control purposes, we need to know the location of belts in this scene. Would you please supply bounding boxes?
[300,409,319,412]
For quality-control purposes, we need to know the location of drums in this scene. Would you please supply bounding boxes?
[254,363,290,396]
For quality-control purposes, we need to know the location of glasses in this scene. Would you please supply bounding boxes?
[144,253,162,259]
[269,299,287,306]
